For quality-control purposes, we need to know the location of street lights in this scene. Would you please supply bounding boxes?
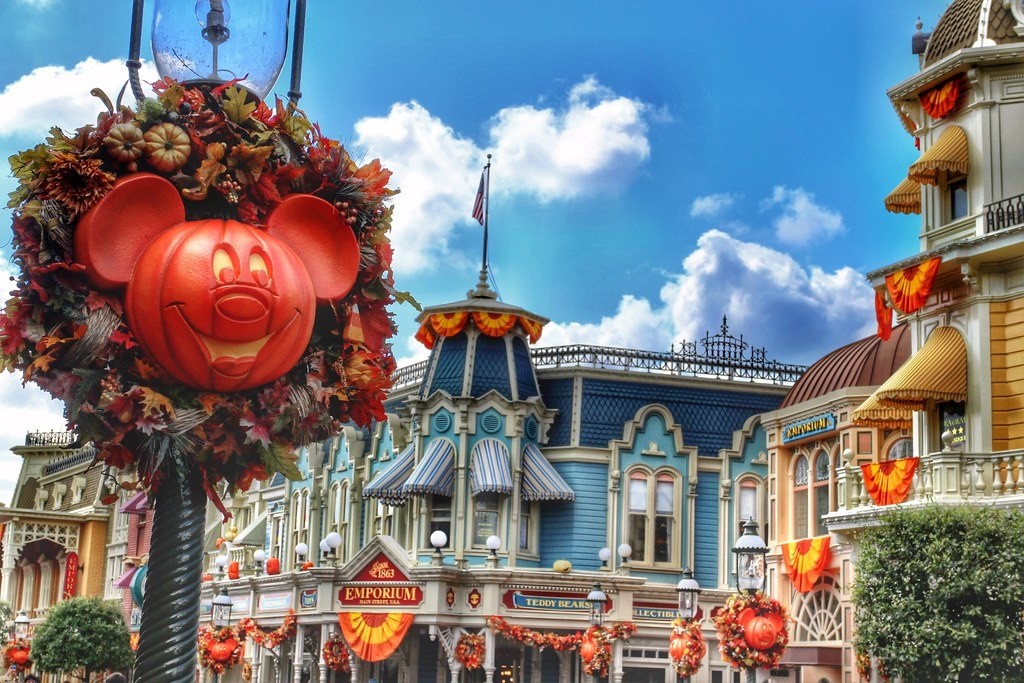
[673,565,703,683]
[209,585,234,683]
[728,515,772,683]
[586,581,609,683]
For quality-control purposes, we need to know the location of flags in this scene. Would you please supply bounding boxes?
[473,169,486,225]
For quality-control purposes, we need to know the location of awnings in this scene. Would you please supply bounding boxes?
[402,438,456,499]
[469,439,514,497]
[112,566,137,589]
[361,442,415,500]
[136,496,157,509]
[908,126,968,185]
[522,443,576,503]
[379,497,406,507]
[883,177,922,215]
[877,325,967,412]
[119,492,147,514]
[851,387,912,430]
[231,510,266,545]
